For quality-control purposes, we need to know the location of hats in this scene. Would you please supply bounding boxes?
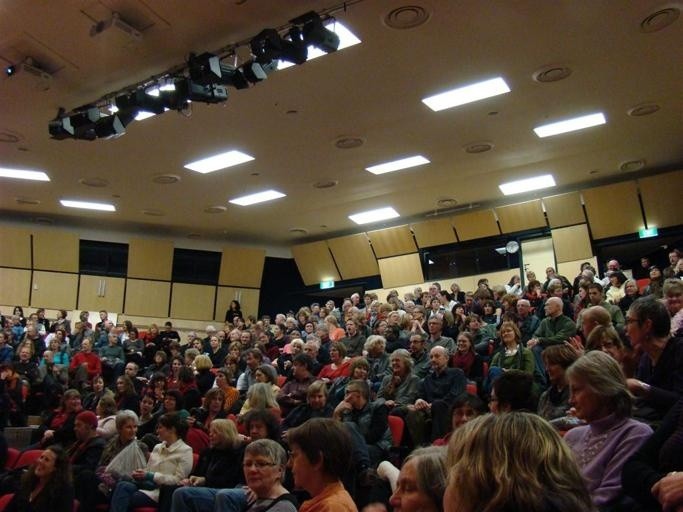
[73,411,98,427]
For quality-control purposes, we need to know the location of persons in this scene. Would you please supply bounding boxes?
[95,310,108,332]
[341,290,366,359]
[447,331,483,382]
[158,419,241,511]
[539,277,574,319]
[469,305,501,327]
[537,344,572,421]
[229,329,242,343]
[96,395,118,439]
[145,372,168,403]
[527,296,577,387]
[618,278,638,315]
[437,290,459,312]
[585,325,637,378]
[0,346,40,428]
[0,365,29,413]
[274,313,286,328]
[4,445,74,512]
[160,322,181,358]
[119,320,133,344]
[487,365,540,415]
[358,334,391,401]
[324,316,346,341]
[207,335,227,369]
[562,351,657,510]
[123,327,144,362]
[227,341,243,351]
[425,317,457,355]
[303,341,320,364]
[136,393,158,439]
[63,410,105,510]
[340,358,373,401]
[371,348,422,410]
[439,305,466,341]
[203,325,217,342]
[182,348,200,368]
[241,331,252,348]
[295,306,312,330]
[169,343,184,358]
[37,308,50,332]
[501,293,518,320]
[231,313,239,327]
[246,383,279,410]
[99,331,126,377]
[503,276,523,297]
[143,351,171,380]
[286,418,358,512]
[352,468,394,512]
[463,313,492,351]
[400,334,430,378]
[32,351,68,397]
[244,313,258,335]
[282,380,335,443]
[215,368,240,412]
[222,354,242,386]
[280,353,319,405]
[523,271,542,308]
[254,365,281,401]
[0,333,15,364]
[279,330,303,347]
[96,410,150,496]
[389,444,447,512]
[111,413,194,511]
[637,255,663,295]
[278,339,305,378]
[70,338,102,380]
[541,267,573,300]
[473,278,498,299]
[114,375,140,415]
[602,259,631,286]
[24,313,46,335]
[192,355,216,396]
[621,398,683,511]
[235,319,246,331]
[572,262,607,291]
[12,316,25,344]
[3,319,18,350]
[215,330,229,351]
[13,307,26,327]
[224,300,242,331]
[190,337,209,358]
[167,356,198,394]
[311,304,319,316]
[488,320,541,405]
[318,307,328,318]
[171,408,282,512]
[242,348,278,391]
[303,322,319,342]
[309,320,330,344]
[80,375,115,410]
[449,282,468,303]
[183,388,202,417]
[75,310,92,333]
[125,361,144,393]
[481,301,496,323]
[569,304,622,357]
[261,315,275,330]
[476,291,485,310]
[31,389,82,445]
[39,337,69,371]
[516,299,540,347]
[575,283,623,341]
[605,273,628,304]
[189,388,226,429]
[443,411,602,511]
[317,341,352,379]
[160,391,190,423]
[450,285,465,303]
[44,325,71,358]
[341,378,393,486]
[48,309,71,337]
[364,288,428,337]
[326,300,340,317]
[284,317,300,334]
[144,324,160,349]
[423,281,439,317]
[15,339,40,365]
[26,325,47,358]
[340,321,367,357]
[242,439,299,511]
[433,393,484,446]
[414,345,466,443]
[258,330,284,350]
[663,249,683,278]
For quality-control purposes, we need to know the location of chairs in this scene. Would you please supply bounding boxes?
[2,448,22,472]
[384,413,406,449]
[228,412,247,436]
[177,429,204,471]
[464,379,477,396]
[0,447,47,511]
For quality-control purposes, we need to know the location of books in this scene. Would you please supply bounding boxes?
[657,278,683,337]
[623,296,683,423]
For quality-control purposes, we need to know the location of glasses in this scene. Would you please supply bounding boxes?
[241,460,277,468]
[622,315,646,325]
[344,390,359,394]
[488,396,499,403]
[407,339,425,345]
[286,449,298,459]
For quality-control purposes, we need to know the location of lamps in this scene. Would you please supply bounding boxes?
[117,88,162,115]
[237,55,277,86]
[48,117,72,142]
[302,22,340,56]
[98,113,126,142]
[191,52,242,87]
[143,85,185,118]
[250,29,307,64]
[174,75,227,109]
[68,105,100,143]
